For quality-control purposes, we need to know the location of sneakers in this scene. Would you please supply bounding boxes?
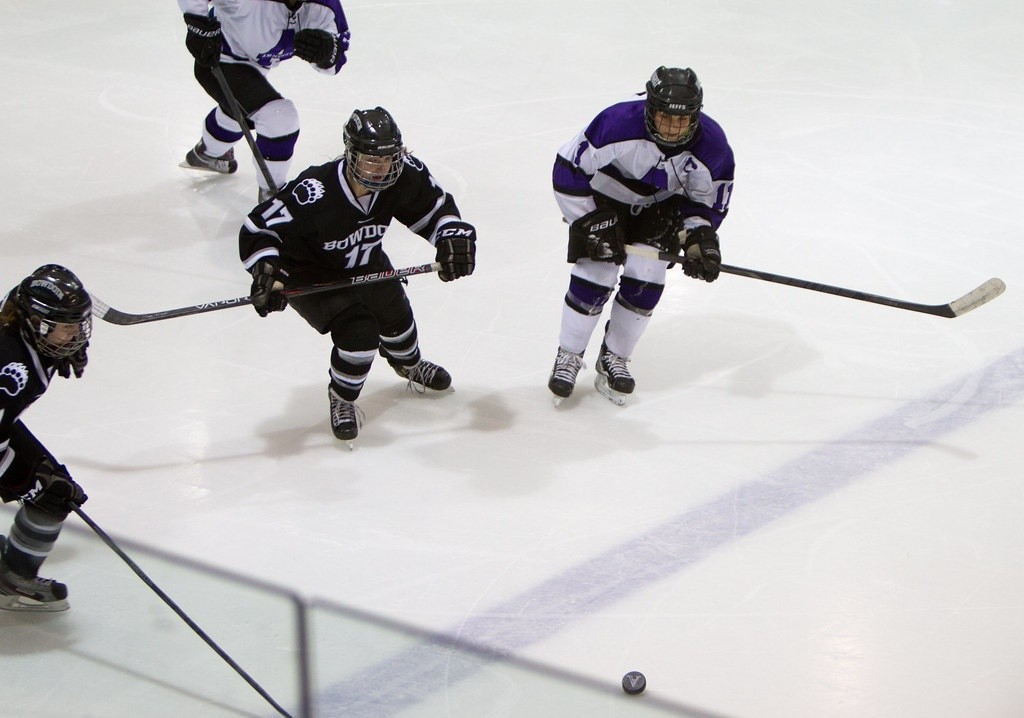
[594,320,635,405]
[178,136,238,174]
[327,383,365,450]
[395,359,455,394]
[548,346,588,407]
[0,534,69,612]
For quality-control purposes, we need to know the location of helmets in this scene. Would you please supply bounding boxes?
[342,107,405,190]
[644,65,702,145]
[17,264,93,358]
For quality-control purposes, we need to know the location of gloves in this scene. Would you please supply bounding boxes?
[184,11,224,68]
[571,202,627,268]
[248,258,292,317]
[680,224,721,282]
[14,454,88,516]
[433,221,477,280]
[291,28,337,71]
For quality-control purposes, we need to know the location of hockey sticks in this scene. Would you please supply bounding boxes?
[83,262,443,327]
[211,60,279,197]
[625,243,1008,321]
[72,501,296,717]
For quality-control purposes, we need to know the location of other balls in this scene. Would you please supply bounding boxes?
[622,671,647,695]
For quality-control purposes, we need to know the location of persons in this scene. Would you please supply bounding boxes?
[239,107,477,451]
[0,264,93,613]
[175,0,350,205]
[548,65,736,408]
